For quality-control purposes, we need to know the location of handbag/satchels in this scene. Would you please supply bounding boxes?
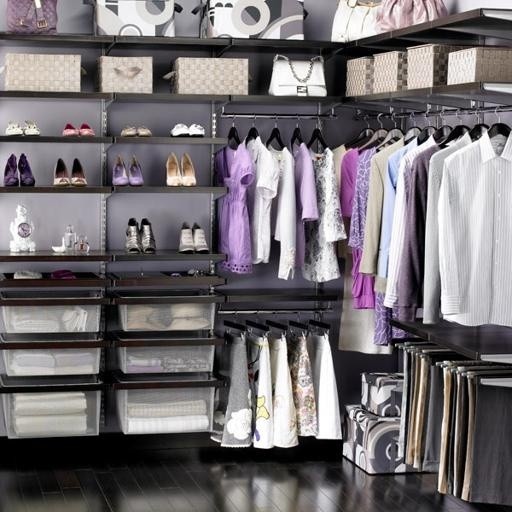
[374,0,450,35]
[267,53,329,98]
[331,0,384,44]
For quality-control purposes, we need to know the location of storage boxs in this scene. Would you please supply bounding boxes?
[357,369,406,419]
[340,402,442,477]
[0,290,219,445]
[342,37,511,99]
[81,0,185,39]
[189,0,310,42]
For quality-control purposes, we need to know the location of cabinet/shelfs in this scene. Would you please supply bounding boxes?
[0,26,231,468]
[331,3,512,115]
[221,30,349,108]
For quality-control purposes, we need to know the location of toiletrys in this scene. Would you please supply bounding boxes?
[75,231,91,253]
[63,223,77,250]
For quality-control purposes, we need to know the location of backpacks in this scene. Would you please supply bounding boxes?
[4,0,59,36]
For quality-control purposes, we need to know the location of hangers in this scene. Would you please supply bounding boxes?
[216,111,333,159]
[339,104,511,162]
[215,302,333,349]
[394,330,512,389]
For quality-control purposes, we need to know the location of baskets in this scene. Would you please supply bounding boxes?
[405,43,512,91]
[345,54,374,97]
[371,50,409,94]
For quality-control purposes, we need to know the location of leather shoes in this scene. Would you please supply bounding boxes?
[191,220,209,254]
[62,123,79,137]
[128,153,145,187]
[111,154,130,187]
[180,152,197,187]
[53,157,71,186]
[137,125,152,137]
[165,151,183,187]
[178,221,195,254]
[23,119,41,136]
[18,153,36,187]
[169,123,189,137]
[125,217,142,254]
[70,158,88,186]
[4,120,24,136]
[3,153,19,187]
[139,217,156,254]
[120,125,138,137]
[79,122,96,137]
[188,122,205,138]
